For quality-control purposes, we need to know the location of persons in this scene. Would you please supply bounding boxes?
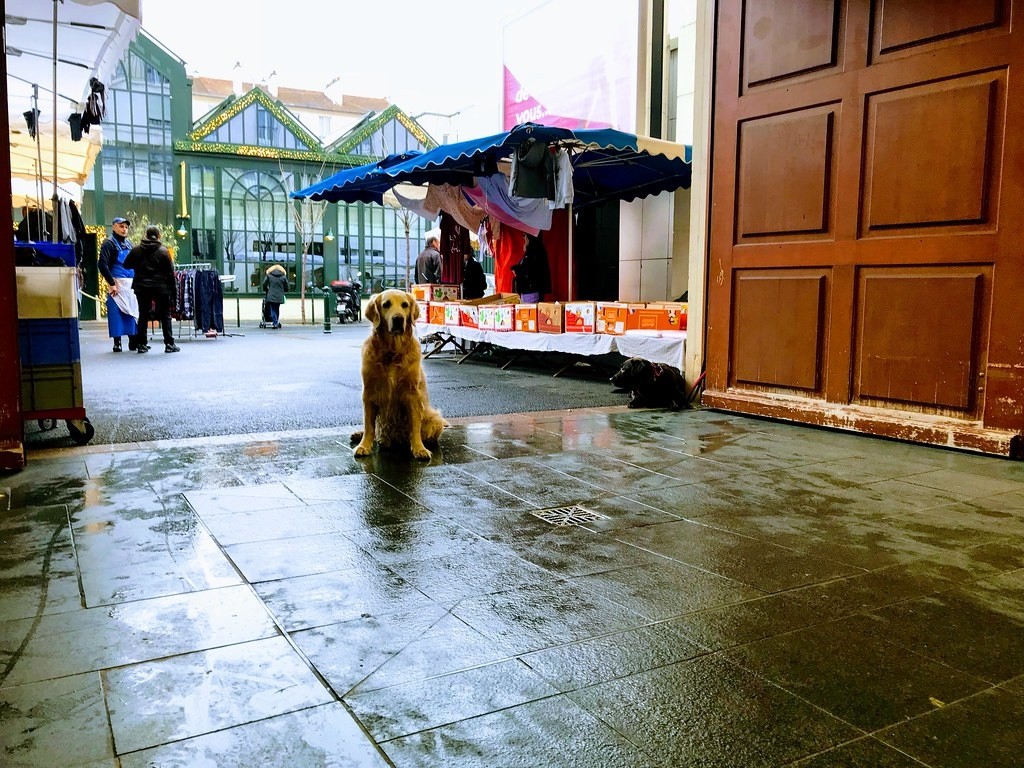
[122,225,180,353]
[98,218,151,352]
[462,246,487,300]
[414,237,441,345]
[262,264,288,329]
[510,229,551,301]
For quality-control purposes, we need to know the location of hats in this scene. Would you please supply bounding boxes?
[112,217,130,226]
[463,246,476,257]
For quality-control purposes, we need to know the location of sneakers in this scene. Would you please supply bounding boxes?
[165,344,180,352]
[138,346,148,353]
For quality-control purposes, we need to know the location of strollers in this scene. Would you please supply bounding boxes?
[259,291,282,329]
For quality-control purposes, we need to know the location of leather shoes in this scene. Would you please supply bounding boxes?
[129,344,151,350]
[113,343,122,351]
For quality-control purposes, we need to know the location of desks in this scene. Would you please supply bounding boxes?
[415,324,686,385]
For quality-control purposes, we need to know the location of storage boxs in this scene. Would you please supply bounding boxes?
[412,283,687,336]
[15,242,82,409]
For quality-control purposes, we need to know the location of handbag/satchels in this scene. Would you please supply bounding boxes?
[520,292,539,303]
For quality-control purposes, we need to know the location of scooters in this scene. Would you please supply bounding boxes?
[330,271,363,324]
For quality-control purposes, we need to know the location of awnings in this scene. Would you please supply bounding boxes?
[5,0,142,211]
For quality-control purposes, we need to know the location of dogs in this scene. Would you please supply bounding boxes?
[608,356,694,411]
[349,288,452,462]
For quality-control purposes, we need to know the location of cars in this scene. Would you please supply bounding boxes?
[374,278,405,293]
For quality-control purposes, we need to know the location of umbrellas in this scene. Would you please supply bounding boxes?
[370,120,693,303]
[288,122,424,302]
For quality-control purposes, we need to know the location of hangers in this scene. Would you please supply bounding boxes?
[174,263,218,272]
[525,128,536,143]
[551,141,561,150]
